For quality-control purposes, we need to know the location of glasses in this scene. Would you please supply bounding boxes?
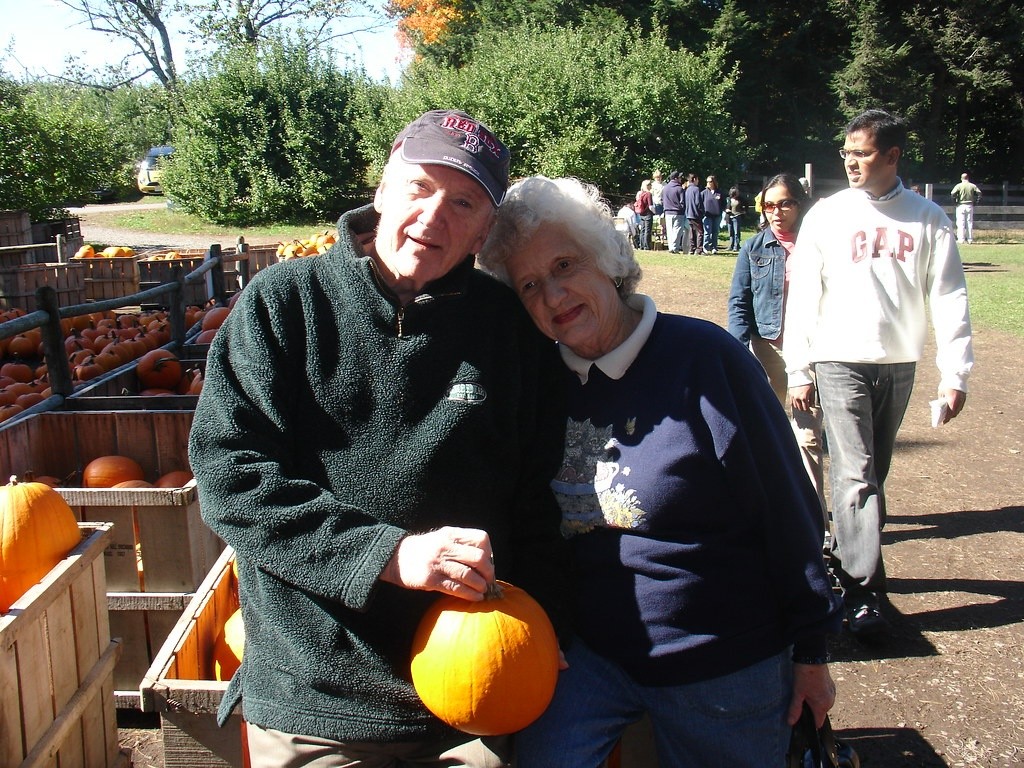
[761,201,799,213]
[839,148,890,161]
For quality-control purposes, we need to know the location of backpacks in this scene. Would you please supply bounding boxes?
[633,192,649,214]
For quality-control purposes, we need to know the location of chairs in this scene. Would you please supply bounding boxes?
[612,217,639,250]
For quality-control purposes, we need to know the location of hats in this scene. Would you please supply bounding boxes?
[670,171,683,180]
[392,109,511,207]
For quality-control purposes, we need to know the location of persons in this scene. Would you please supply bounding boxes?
[616,169,811,255]
[782,110,974,628]
[474,176,835,768]
[950,173,982,244]
[727,174,835,561]
[187,110,560,768]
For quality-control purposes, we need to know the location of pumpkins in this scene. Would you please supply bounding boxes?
[409,579,559,735]
[210,557,245,681]
[0,230,338,616]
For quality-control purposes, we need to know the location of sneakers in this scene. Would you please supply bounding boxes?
[846,587,891,639]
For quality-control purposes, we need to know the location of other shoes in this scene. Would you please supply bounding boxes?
[733,248,738,252]
[707,250,711,255]
[713,249,716,254]
[725,247,733,251]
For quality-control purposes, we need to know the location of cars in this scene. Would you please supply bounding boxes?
[136,144,175,196]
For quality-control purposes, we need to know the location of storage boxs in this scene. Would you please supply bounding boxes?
[0,211,280,768]
